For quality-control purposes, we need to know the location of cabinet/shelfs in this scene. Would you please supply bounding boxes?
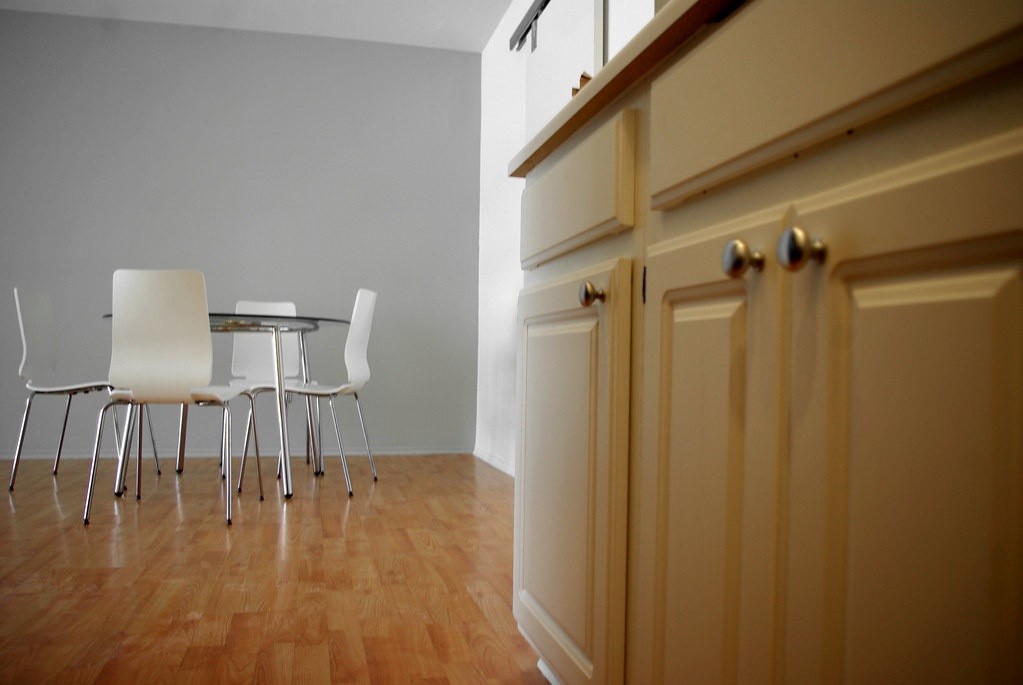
[509,0,1023,685]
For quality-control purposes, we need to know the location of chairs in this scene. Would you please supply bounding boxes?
[6,268,381,529]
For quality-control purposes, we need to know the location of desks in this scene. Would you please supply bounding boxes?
[102,312,353,501]
